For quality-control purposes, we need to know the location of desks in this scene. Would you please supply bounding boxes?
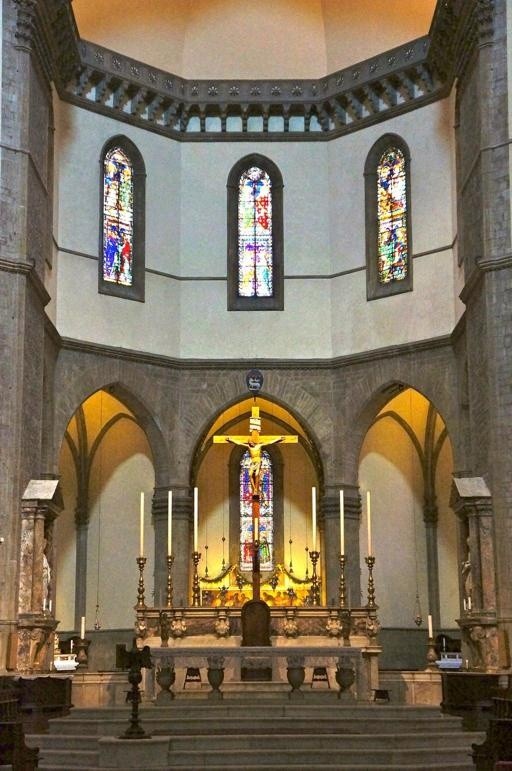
[440,651,462,660]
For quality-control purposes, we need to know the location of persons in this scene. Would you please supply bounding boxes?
[226,436,285,495]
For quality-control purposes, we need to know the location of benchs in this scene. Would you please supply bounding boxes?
[1,674,76,771]
[467,695,512,771]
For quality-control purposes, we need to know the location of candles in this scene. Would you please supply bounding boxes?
[443,637,445,647]
[311,486,317,552]
[43,599,46,612]
[254,518,259,543]
[467,597,471,611]
[427,614,433,640]
[167,490,172,557]
[80,617,86,641]
[366,491,373,559]
[138,492,144,558]
[49,600,53,614]
[193,486,198,554]
[339,490,345,557]
[463,599,466,611]
